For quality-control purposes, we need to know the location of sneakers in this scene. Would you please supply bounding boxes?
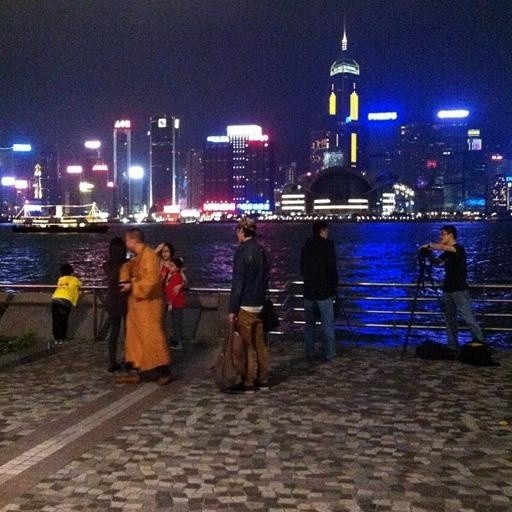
[256,383,269,390]
[119,374,140,383]
[158,368,172,385]
[230,384,255,393]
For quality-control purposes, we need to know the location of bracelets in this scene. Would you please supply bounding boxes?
[428,242,431,248]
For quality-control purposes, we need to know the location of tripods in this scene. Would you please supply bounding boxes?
[400,259,460,361]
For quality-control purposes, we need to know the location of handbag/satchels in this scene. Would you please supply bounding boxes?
[211,321,246,393]
[261,300,279,331]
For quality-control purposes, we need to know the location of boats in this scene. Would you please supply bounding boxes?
[12,201,110,233]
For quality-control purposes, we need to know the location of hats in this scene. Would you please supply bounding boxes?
[232,218,256,233]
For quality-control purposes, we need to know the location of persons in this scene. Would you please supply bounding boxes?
[299,221,340,361]
[226,220,271,393]
[117,228,174,386]
[101,237,131,374]
[151,241,188,346]
[418,225,483,347]
[166,258,187,350]
[51,264,83,345]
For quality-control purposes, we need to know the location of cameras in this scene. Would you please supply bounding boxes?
[418,247,434,259]
